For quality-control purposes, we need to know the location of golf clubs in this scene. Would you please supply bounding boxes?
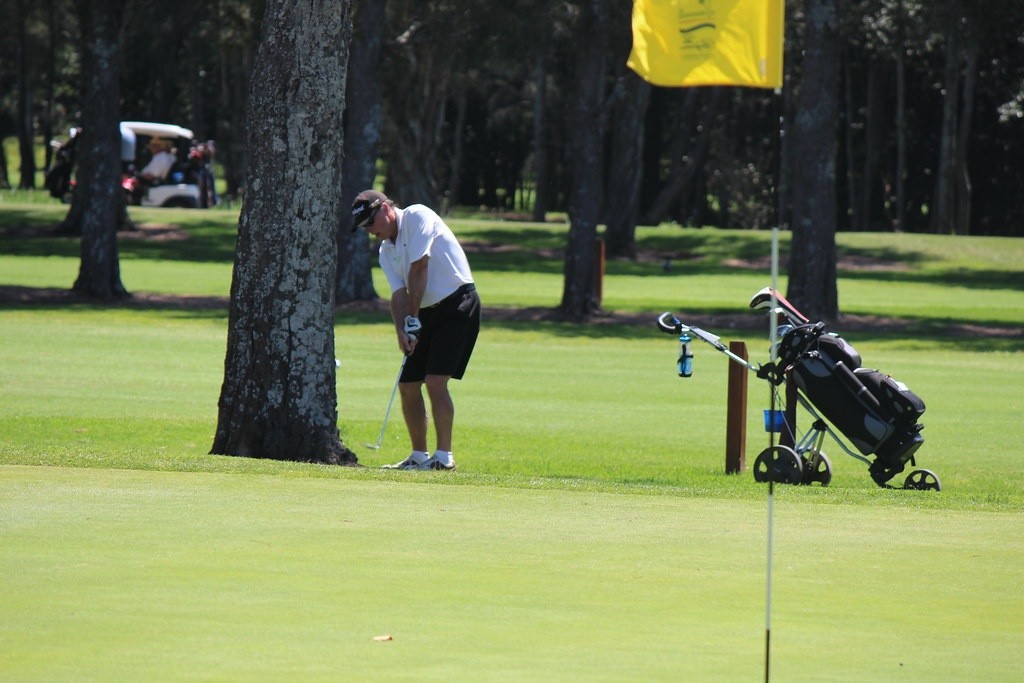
[365,341,414,450]
[749,286,828,363]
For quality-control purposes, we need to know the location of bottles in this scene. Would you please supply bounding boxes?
[677,333,694,377]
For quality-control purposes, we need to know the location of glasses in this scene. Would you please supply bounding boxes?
[363,206,381,228]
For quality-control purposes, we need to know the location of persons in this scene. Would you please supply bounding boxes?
[351,191,482,472]
[136,138,170,202]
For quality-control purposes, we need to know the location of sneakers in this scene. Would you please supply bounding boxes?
[404,456,455,472]
[382,458,418,469]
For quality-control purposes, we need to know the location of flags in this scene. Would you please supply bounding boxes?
[623,0,785,90]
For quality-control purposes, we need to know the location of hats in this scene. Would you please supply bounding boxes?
[348,189,387,235]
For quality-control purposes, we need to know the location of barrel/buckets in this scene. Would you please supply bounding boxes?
[763,390,785,432]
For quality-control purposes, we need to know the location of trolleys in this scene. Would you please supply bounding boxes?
[657,284,944,492]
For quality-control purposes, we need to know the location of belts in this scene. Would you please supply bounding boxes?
[419,282,475,313]
